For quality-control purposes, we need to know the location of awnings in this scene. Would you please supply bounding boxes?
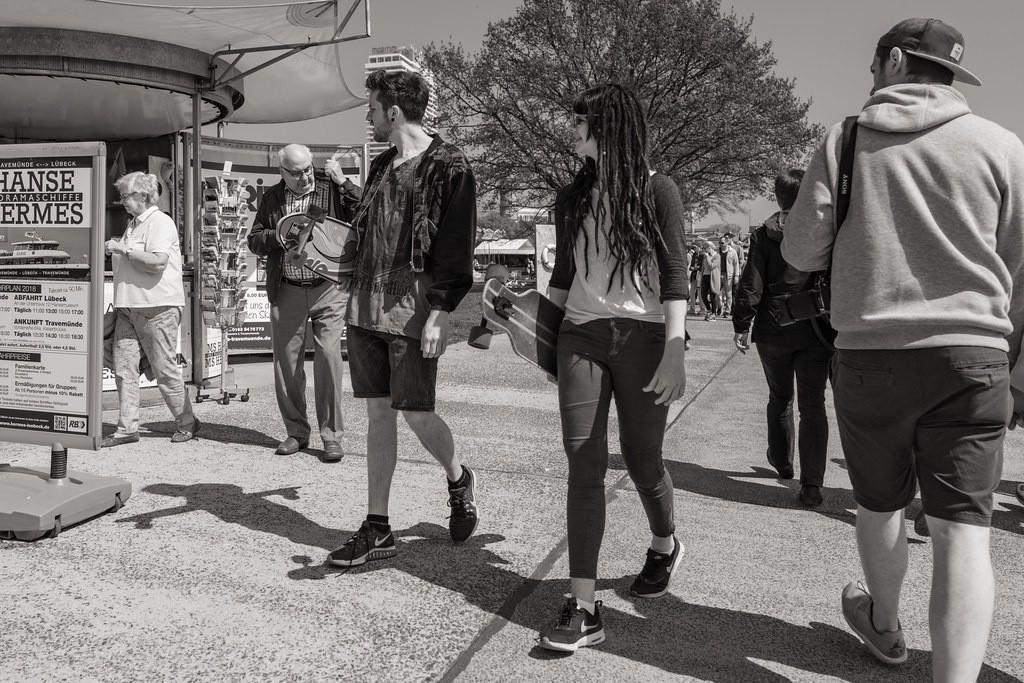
[0,0,372,123]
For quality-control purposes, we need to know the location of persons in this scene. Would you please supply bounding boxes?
[101,172,202,447]
[328,68,480,566]
[780,19,1024,683]
[686,232,750,317]
[731,170,839,507]
[701,241,722,322]
[541,82,688,652]
[248,143,363,460]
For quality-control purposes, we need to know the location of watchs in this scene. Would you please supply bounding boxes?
[126,247,133,258]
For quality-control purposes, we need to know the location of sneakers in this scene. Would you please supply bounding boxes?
[328,520,396,579]
[629,532,685,598]
[841,580,907,662]
[443,463,479,544]
[538,596,605,653]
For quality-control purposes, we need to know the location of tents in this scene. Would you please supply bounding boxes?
[474,239,535,265]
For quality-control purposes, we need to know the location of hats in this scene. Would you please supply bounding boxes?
[692,242,701,248]
[877,17,983,87]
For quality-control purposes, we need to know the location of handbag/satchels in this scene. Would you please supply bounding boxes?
[103,303,144,378]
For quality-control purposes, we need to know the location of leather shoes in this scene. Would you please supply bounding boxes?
[277,434,310,454]
[324,439,344,460]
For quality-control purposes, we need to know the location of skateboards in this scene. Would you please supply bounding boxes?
[274,201,363,289]
[467,263,566,385]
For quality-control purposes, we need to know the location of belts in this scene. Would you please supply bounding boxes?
[280,277,326,289]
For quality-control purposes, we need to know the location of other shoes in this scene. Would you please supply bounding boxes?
[1015,483,1024,506]
[766,446,794,479]
[100,431,139,447]
[686,307,733,322]
[170,417,202,443]
[798,483,823,507]
[914,505,931,537]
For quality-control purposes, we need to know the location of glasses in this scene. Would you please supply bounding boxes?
[719,242,724,244]
[570,113,600,128]
[282,163,312,178]
[120,191,137,201]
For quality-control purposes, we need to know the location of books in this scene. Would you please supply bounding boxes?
[200,175,250,326]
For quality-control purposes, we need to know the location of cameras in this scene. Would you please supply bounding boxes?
[764,272,831,328]
